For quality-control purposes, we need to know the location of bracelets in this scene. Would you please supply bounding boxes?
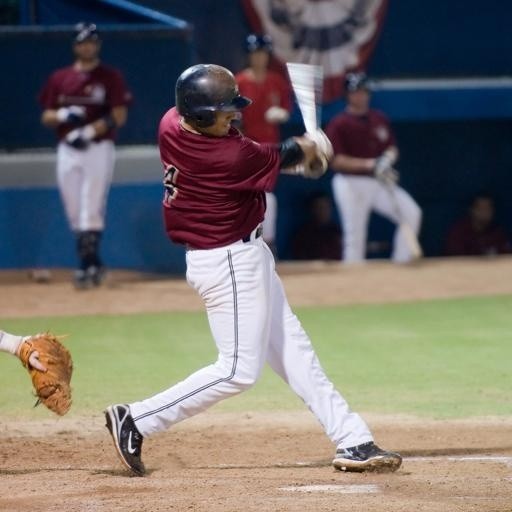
[83,124,97,139]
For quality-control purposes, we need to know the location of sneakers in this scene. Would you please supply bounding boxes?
[73,268,86,289]
[86,264,102,288]
[101,400,150,478]
[330,441,404,473]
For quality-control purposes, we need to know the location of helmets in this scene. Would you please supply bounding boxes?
[174,62,253,128]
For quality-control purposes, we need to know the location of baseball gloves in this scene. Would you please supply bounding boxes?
[19,331,72,415]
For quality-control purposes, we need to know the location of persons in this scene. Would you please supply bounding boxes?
[435,193,512,256]
[103,65,402,476]
[38,24,134,288]
[293,194,341,260]
[233,33,292,244]
[0,329,74,416]
[324,71,424,266]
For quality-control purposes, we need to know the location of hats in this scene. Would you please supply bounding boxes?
[343,71,376,92]
[68,19,100,43]
[241,31,272,54]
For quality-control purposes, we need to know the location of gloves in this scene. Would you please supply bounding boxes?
[303,129,333,161]
[373,150,401,190]
[65,125,96,148]
[294,152,329,181]
[56,105,88,125]
